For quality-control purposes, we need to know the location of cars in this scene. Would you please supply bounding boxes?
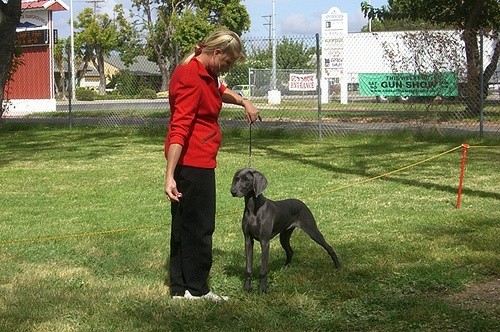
[232,84,257,97]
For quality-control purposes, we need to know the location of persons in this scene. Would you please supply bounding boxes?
[163,31,263,302]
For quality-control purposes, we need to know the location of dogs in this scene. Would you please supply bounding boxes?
[230,168,341,295]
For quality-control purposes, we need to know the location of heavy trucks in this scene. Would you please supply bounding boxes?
[345,29,500,103]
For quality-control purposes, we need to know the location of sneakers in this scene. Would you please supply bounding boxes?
[171,290,228,302]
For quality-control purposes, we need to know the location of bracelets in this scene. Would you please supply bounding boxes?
[241,98,248,107]
